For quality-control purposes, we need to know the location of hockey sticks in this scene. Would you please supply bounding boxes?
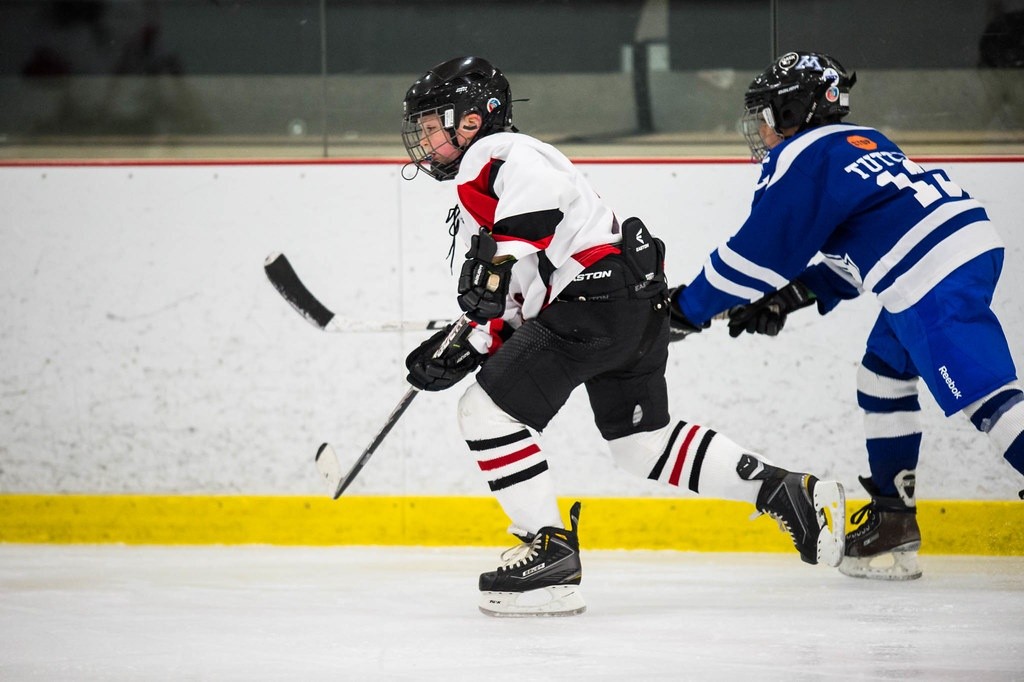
[313,310,473,501]
[261,250,783,334]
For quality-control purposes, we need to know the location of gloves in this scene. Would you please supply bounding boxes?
[456,230,518,325]
[405,321,489,392]
[727,283,810,338]
[668,283,712,344]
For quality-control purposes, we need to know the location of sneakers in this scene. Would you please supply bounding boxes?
[736,453,845,568]
[478,501,588,619]
[838,475,923,582]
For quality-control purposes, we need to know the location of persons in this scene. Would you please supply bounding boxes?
[400,50,848,616]
[668,54,1024,577]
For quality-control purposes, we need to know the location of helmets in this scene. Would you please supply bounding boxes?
[741,51,859,164]
[401,55,530,182]
